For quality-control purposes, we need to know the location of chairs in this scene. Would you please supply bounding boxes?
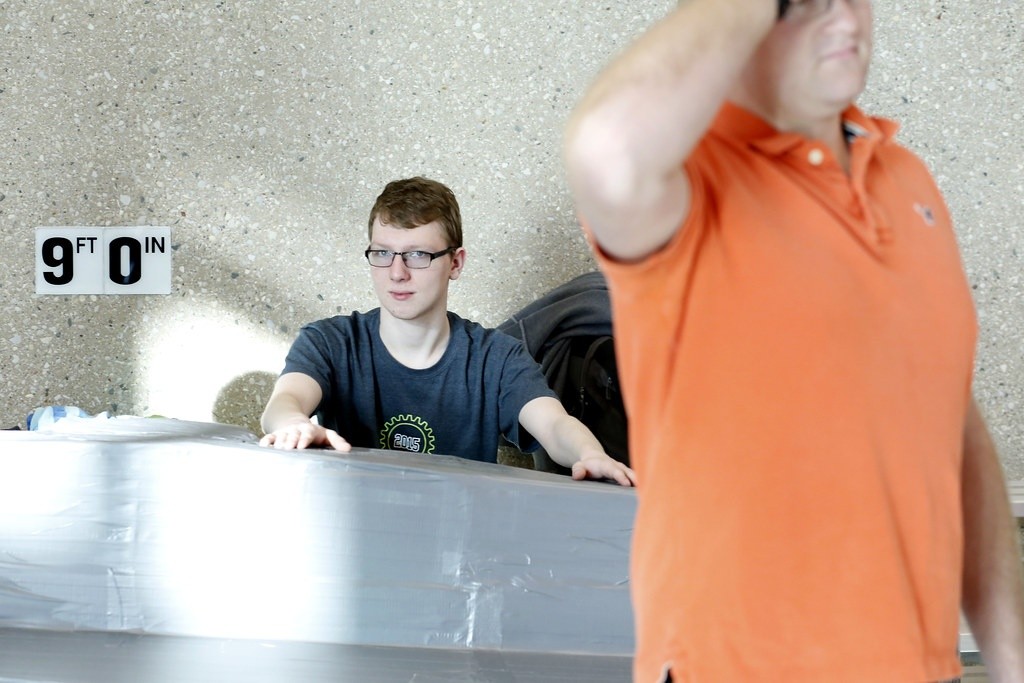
[492,272,630,483]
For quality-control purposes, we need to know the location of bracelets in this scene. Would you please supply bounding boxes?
[776,0,788,20]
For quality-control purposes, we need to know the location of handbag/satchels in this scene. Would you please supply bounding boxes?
[557,336,630,476]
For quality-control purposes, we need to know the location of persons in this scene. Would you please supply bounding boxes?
[563,1,1022,682]
[257,176,638,487]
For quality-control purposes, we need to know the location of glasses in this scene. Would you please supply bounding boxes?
[784,0,832,25]
[366,245,459,269]
